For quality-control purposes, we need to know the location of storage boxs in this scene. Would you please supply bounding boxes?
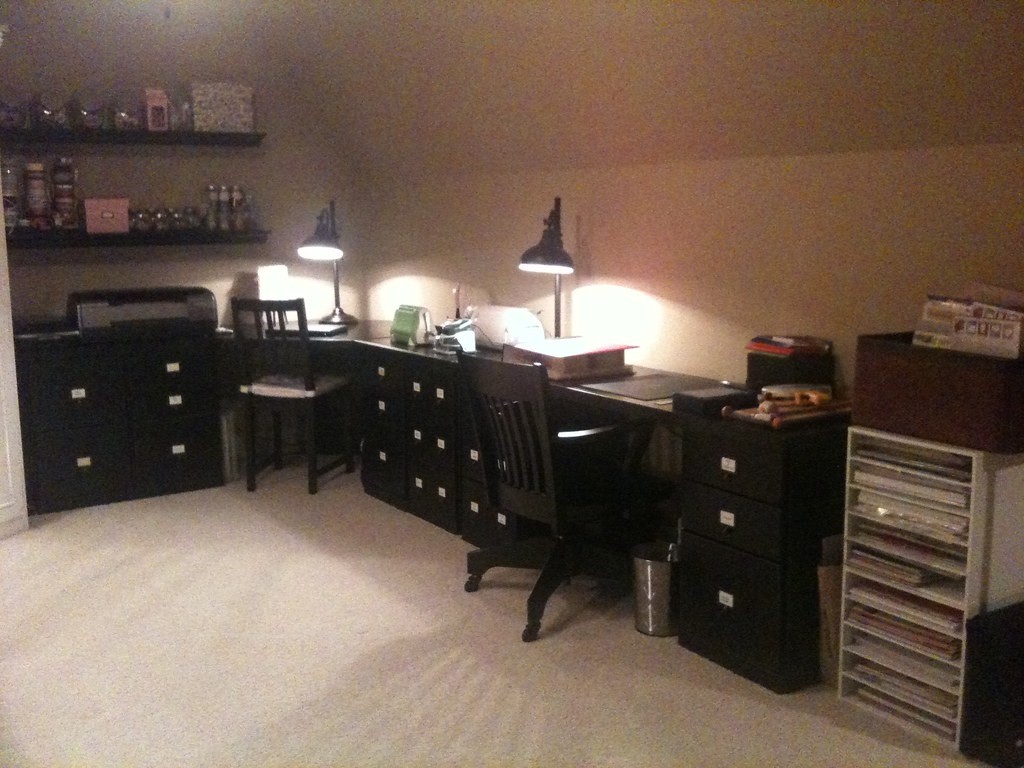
[189,82,256,130]
[76,198,129,235]
[748,351,835,391]
[851,331,1023,456]
[958,600,1024,767]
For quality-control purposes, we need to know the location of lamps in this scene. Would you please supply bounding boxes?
[298,200,358,326]
[519,194,574,340]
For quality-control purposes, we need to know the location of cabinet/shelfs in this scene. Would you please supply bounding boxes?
[13,318,227,518]
[0,129,269,249]
[837,428,1024,750]
[350,340,847,694]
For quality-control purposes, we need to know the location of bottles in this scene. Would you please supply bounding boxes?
[128,183,247,232]
[1,156,77,227]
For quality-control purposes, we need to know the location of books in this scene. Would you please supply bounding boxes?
[845,442,974,740]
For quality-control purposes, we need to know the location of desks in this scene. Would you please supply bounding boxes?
[218,321,396,456]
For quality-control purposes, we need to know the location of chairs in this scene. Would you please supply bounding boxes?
[231,297,355,494]
[457,348,673,645]
